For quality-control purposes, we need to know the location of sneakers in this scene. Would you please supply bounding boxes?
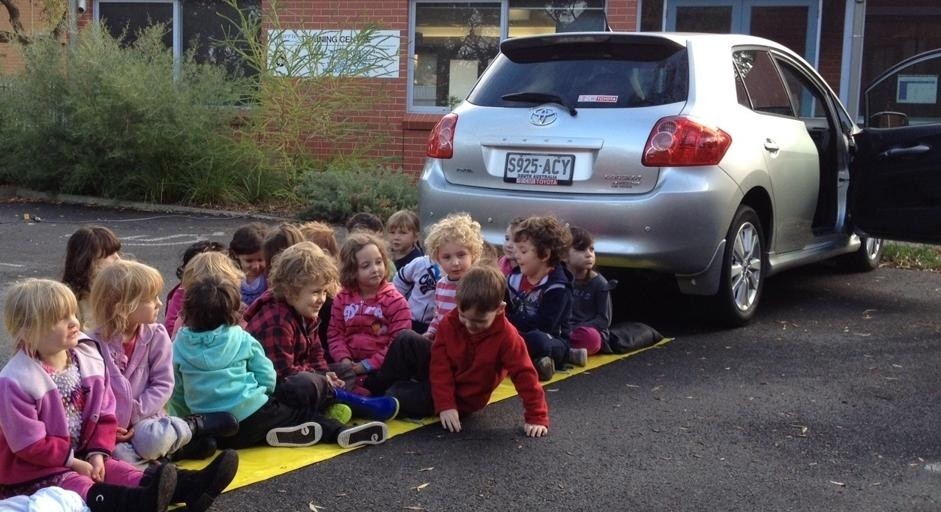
[570,344,588,367]
[266,422,323,447]
[337,420,391,450]
[536,356,555,381]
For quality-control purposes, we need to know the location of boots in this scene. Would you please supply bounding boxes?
[85,464,177,511]
[167,439,219,462]
[184,411,240,438]
[334,389,399,424]
[139,448,239,511]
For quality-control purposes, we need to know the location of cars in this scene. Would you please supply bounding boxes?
[418,31,941,323]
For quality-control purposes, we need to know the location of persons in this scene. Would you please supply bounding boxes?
[165,252,246,339]
[263,223,306,274]
[422,211,483,340]
[347,213,384,235]
[166,240,242,316]
[386,209,424,269]
[0,278,239,512]
[392,255,446,333]
[227,223,270,304]
[61,226,122,330]
[299,221,339,264]
[243,241,399,422]
[86,261,237,471]
[497,217,528,276]
[561,227,618,356]
[172,276,389,449]
[327,234,412,395]
[363,265,549,437]
[504,216,588,381]
